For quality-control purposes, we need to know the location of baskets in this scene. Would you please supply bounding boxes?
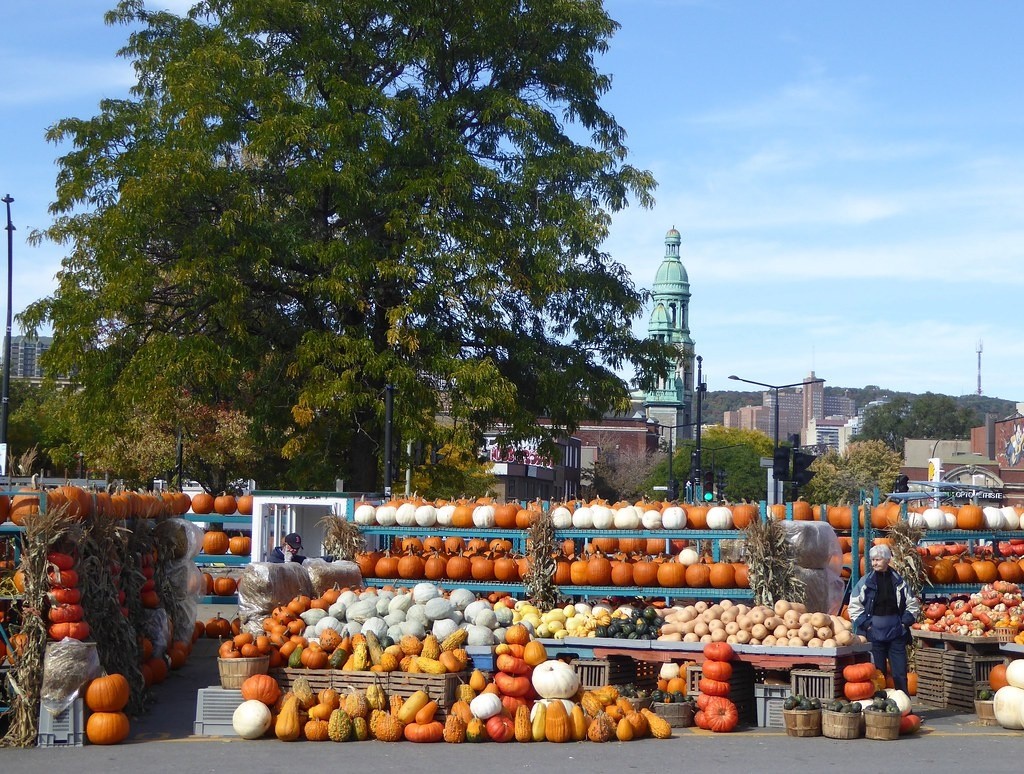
[995,627,1018,643]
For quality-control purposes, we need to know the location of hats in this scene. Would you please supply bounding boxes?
[285,533,304,549]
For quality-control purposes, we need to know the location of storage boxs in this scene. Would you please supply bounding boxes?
[33,625,1024,745]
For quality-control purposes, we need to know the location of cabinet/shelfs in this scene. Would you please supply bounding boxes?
[340,488,1024,603]
[0,489,104,713]
[185,513,285,605]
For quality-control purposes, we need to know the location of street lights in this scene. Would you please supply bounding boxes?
[728,375,827,504]
[0,193,18,479]
[646,421,708,501]
[685,442,746,472]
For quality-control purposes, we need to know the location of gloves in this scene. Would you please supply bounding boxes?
[284,551,293,564]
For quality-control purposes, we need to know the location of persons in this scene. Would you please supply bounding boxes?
[267,534,304,563]
[954,478,962,484]
[848,544,924,722]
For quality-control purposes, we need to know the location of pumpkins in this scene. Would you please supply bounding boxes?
[217,580,795,744]
[765,494,1024,737]
[352,491,762,530]
[0,472,254,746]
[354,535,756,588]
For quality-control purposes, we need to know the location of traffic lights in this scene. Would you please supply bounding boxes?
[704,470,714,501]
[716,468,728,501]
[792,450,817,487]
